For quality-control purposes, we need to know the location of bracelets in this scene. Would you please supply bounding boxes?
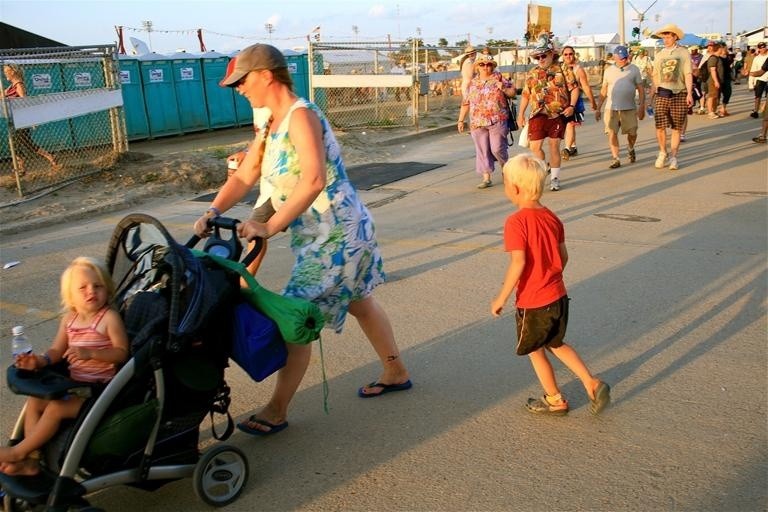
[40,352,52,366]
[204,208,220,218]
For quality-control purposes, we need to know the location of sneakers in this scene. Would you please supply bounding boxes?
[680,134,687,142]
[654,152,669,167]
[550,175,561,192]
[687,105,759,119]
[477,179,492,190]
[752,136,767,143]
[627,148,637,162]
[669,158,680,170]
[560,143,577,162]
[609,158,621,168]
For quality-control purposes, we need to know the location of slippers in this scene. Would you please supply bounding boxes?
[235,412,289,437]
[357,375,414,397]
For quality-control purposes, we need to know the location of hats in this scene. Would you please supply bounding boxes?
[473,54,498,70]
[218,43,285,87]
[614,45,630,59]
[464,46,492,56]
[689,40,767,50]
[654,23,685,40]
[530,43,554,55]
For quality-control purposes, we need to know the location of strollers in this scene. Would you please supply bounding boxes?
[1,204,276,512]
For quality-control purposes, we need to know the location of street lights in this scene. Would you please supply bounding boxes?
[414,24,424,46]
[352,23,360,43]
[262,22,276,50]
[652,14,663,33]
[139,20,154,57]
[483,25,495,45]
[572,21,582,38]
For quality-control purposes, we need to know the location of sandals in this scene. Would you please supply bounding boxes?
[524,392,570,417]
[587,381,611,417]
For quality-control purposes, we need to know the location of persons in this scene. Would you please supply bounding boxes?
[1,61,60,178]
[458,25,768,191]
[195,42,413,435]
[0,256,128,476]
[490,153,609,416]
[324,58,461,107]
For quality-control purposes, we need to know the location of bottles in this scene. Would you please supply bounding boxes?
[11,326,38,372]
[645,104,654,118]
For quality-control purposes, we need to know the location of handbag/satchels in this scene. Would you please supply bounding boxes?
[497,71,519,132]
[518,119,529,148]
